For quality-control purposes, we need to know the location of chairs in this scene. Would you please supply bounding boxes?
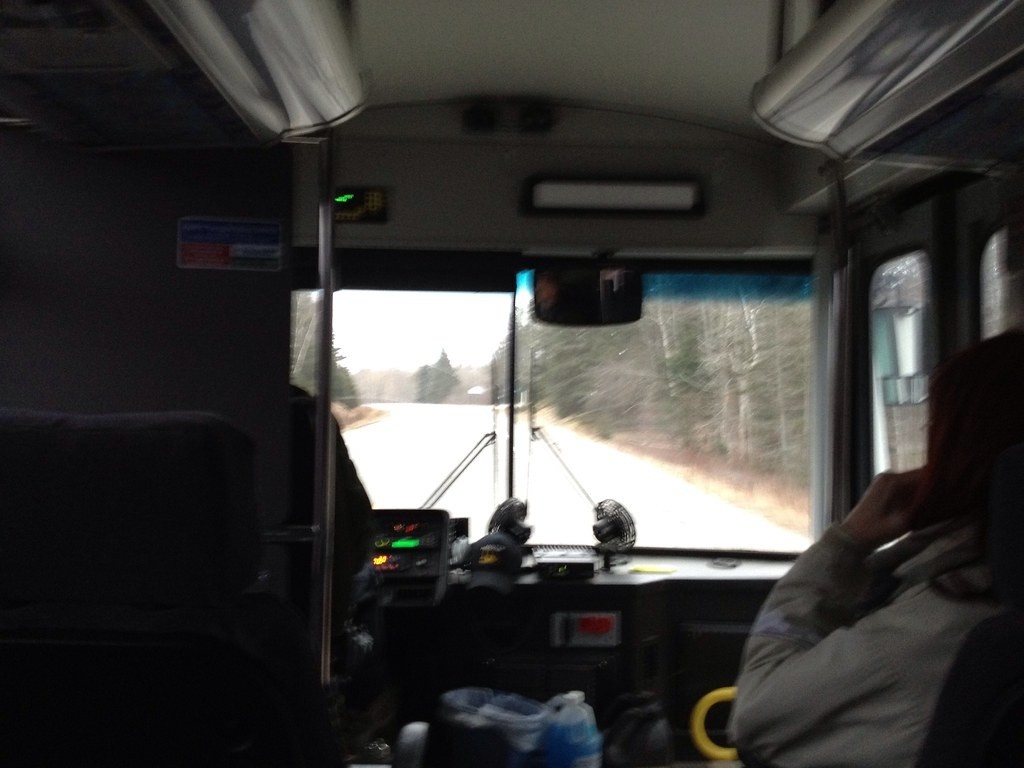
[916,443,1024,768]
[293,397,395,748]
[0,410,341,768]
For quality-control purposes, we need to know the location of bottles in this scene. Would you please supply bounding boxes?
[550,691,603,768]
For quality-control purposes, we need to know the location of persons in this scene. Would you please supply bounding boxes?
[290,384,379,678]
[727,325,1024,768]
[534,273,569,326]
